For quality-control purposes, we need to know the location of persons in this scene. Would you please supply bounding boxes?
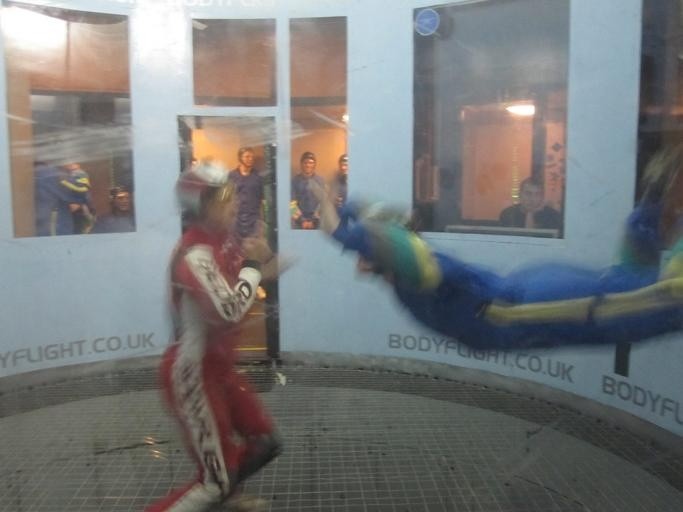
[141,159,284,510]
[500,176,562,240]
[88,184,136,233]
[290,151,326,230]
[304,141,683,354]
[227,146,270,300]
[332,154,347,210]
[31,129,92,236]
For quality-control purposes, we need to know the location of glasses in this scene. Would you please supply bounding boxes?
[204,184,236,202]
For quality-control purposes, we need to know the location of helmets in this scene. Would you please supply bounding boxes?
[340,155,348,165]
[109,186,129,201]
[176,165,228,215]
[238,147,253,161]
[301,152,316,161]
